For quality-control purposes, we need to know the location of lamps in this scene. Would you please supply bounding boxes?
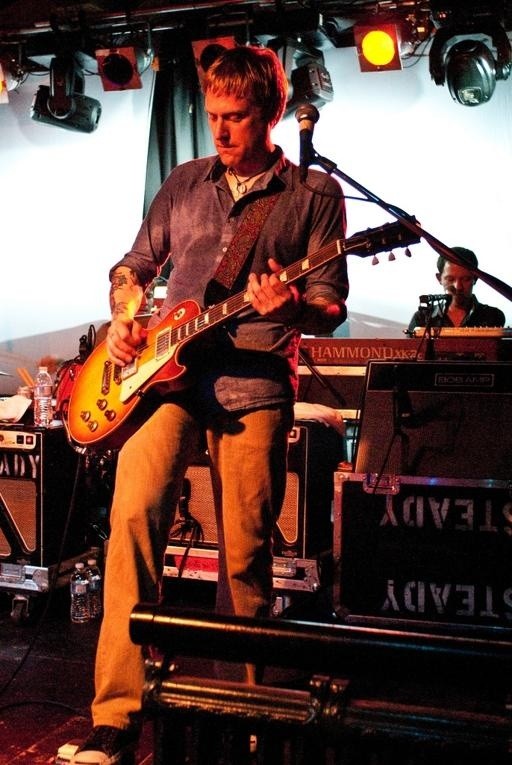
[0,14,143,136]
[350,23,409,75]
[418,3,510,109]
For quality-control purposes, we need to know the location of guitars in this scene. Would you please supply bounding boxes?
[67,214,420,450]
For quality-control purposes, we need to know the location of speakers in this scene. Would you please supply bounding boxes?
[0,427,86,565]
[163,426,320,553]
[357,359,512,478]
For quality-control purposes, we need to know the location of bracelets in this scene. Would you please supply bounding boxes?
[284,297,309,330]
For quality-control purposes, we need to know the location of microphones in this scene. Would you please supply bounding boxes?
[391,363,412,419]
[295,101,320,184]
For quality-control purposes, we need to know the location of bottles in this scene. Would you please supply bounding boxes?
[33,365,57,430]
[70,558,104,625]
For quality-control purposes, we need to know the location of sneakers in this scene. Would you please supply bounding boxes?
[71,722,134,765]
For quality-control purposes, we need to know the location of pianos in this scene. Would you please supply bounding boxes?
[297,336,512,367]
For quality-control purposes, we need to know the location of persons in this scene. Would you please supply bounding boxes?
[71,47,350,765]
[403,245,507,338]
